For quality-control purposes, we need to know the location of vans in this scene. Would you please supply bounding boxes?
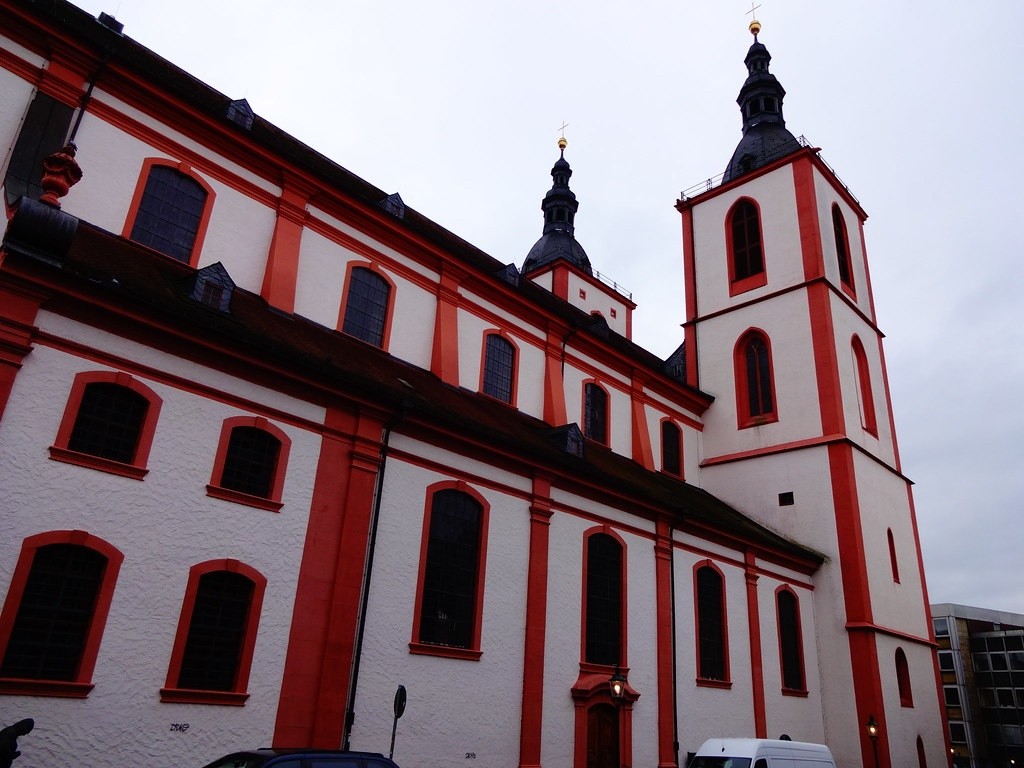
[689,736,834,768]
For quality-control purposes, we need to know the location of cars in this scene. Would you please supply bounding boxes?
[202,747,399,768]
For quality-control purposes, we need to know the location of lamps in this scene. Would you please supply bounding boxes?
[608,667,626,706]
